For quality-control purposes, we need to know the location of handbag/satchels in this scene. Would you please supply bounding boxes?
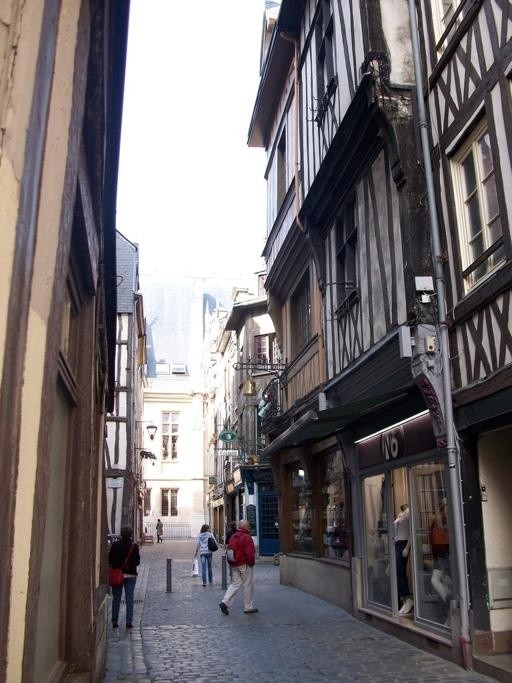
[208,537,218,551]
[107,567,124,586]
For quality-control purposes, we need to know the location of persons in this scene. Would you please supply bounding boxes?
[218,519,259,614]
[428,495,455,625]
[224,520,242,576]
[155,519,163,542]
[108,523,140,627]
[394,503,415,614]
[194,523,217,585]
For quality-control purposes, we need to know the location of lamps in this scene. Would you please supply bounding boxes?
[140,420,158,441]
[233,356,286,396]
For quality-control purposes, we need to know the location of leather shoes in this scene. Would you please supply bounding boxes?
[220,603,229,615]
[113,621,118,628]
[126,623,133,628]
[244,609,258,613]
